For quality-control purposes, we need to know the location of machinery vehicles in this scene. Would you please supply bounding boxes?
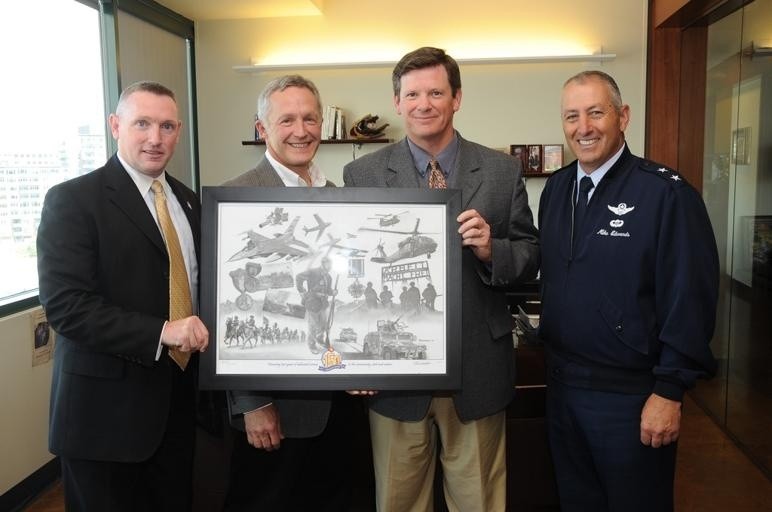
[362,313,427,361]
[339,327,357,342]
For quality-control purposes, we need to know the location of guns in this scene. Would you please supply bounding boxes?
[324,275,340,350]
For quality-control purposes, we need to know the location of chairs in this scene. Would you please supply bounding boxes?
[736,215,771,305]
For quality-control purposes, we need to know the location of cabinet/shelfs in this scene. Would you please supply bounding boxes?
[242,141,554,177]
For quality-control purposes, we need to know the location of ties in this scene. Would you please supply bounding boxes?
[573,175,595,246]
[151,180,194,373]
[428,160,447,190]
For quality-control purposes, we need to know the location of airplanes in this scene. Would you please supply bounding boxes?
[226,207,439,268]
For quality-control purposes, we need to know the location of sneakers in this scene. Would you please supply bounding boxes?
[310,343,326,354]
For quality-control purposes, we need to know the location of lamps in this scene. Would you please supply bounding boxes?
[743,41,772,60]
[232,46,617,80]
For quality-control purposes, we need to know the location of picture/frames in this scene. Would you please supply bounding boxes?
[730,128,751,165]
[509,144,564,173]
[194,186,461,391]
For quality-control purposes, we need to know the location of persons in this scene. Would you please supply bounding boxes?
[345,46,541,511]
[231,256,437,355]
[538,70,719,512]
[222,75,358,511]
[36,81,202,512]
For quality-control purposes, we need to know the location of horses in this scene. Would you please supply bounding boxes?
[224,316,274,349]
